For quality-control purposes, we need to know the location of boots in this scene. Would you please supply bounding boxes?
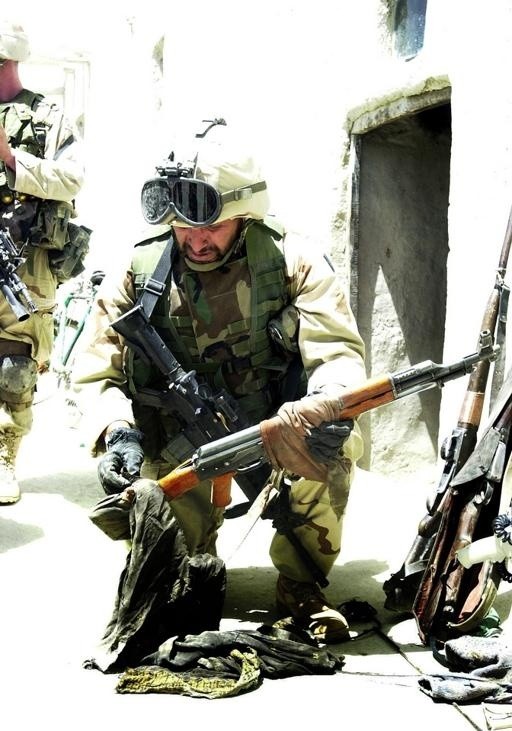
[272,573,352,640]
[0,434,20,504]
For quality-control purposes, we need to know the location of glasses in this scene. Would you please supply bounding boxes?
[140,177,242,227]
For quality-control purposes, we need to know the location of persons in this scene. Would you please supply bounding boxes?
[63,142,378,646]
[0,21,95,508]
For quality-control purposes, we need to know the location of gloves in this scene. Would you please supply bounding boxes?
[99,431,148,496]
[306,418,354,466]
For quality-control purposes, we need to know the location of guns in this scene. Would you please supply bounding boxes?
[1,213,37,321]
[412,393,511,652]
[384,212,512,615]
[112,305,329,589]
[90,350,504,541]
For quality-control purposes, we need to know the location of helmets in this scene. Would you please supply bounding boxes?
[148,137,270,230]
[0,18,29,63]
